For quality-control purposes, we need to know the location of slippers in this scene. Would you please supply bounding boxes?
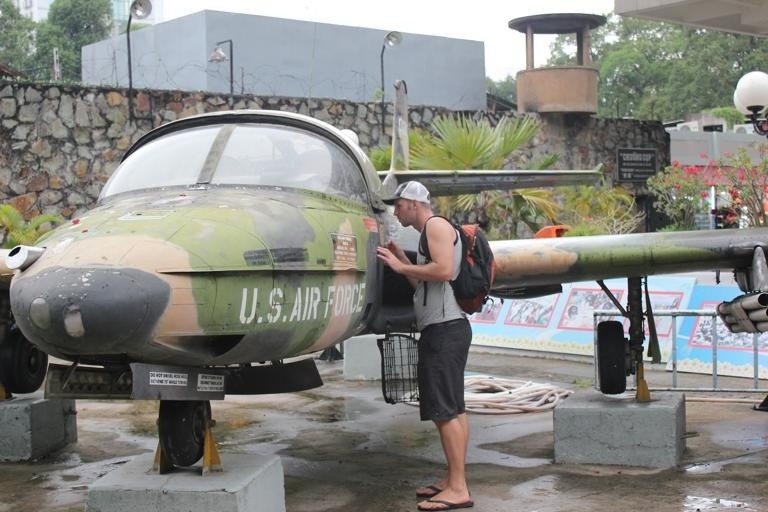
[416,486,442,499]
[417,500,472,512]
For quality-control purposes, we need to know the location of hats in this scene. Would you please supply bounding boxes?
[380,180,430,205]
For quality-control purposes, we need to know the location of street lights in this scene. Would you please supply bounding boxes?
[209,40,235,94]
[125,1,154,90]
[379,30,402,106]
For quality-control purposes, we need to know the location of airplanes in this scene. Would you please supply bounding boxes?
[0,76,767,470]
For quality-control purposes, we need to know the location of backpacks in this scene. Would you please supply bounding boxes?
[418,215,496,315]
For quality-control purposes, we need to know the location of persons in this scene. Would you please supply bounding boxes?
[724,189,742,228]
[757,186,768,226]
[375,179,474,511]
